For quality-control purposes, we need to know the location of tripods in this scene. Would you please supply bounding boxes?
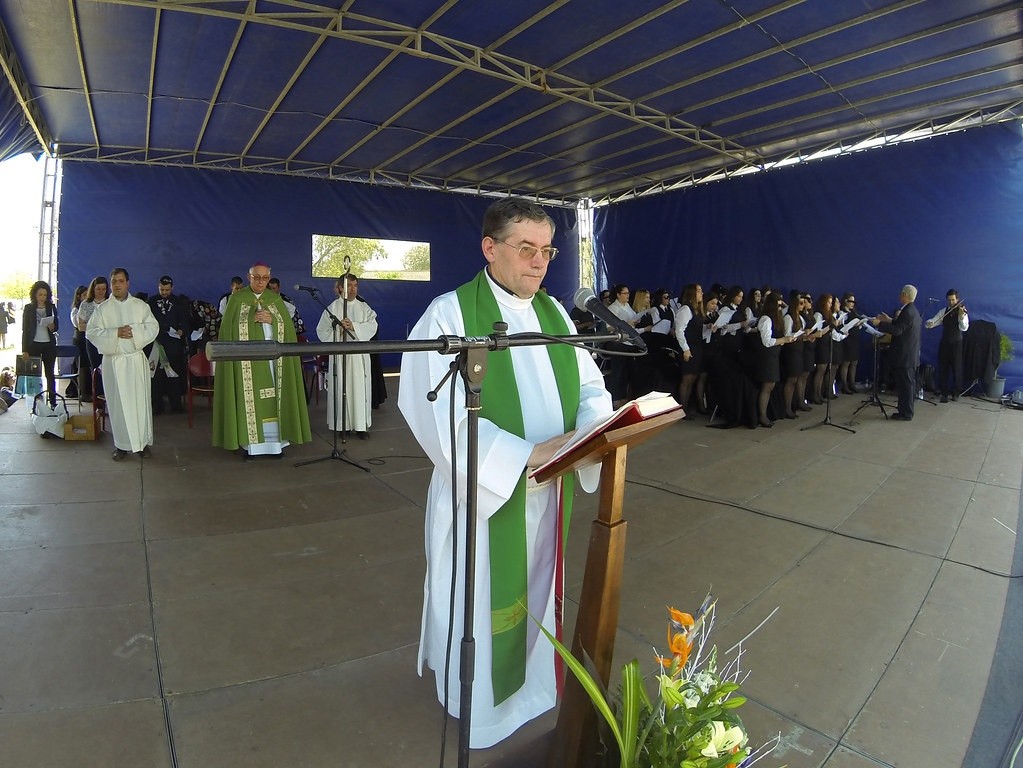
[800,325,899,434]
[294,291,370,472]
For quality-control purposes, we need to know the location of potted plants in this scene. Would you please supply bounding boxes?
[987,334,1016,398]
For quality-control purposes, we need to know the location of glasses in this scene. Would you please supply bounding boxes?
[494,236,559,261]
[661,295,670,300]
[249,274,270,283]
[621,292,631,295]
[776,304,782,308]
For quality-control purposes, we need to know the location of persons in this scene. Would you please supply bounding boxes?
[0,261,390,459]
[540,282,924,430]
[926,288,969,403]
[399,196,613,751]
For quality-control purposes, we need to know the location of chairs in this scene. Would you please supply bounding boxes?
[47,343,216,429]
[295,336,330,407]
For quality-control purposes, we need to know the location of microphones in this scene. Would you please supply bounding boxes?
[574,288,647,349]
[294,285,320,291]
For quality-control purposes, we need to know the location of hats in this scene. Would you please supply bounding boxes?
[254,262,268,266]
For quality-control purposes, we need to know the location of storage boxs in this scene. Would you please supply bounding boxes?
[64,414,101,440]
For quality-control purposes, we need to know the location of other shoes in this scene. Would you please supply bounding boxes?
[245,449,256,463]
[112,447,126,462]
[342,431,351,439]
[138,447,152,458]
[890,412,913,421]
[359,431,370,439]
[696,406,710,415]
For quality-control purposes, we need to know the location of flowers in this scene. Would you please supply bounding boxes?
[511,582,790,768]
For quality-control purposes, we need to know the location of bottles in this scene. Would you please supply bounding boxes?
[918,386,923,399]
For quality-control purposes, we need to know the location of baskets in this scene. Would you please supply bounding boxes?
[31,390,69,440]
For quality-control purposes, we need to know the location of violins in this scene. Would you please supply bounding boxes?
[953,302,968,315]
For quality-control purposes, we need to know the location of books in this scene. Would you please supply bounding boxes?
[528,390,683,479]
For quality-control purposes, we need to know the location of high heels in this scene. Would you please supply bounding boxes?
[784,410,799,419]
[800,403,813,411]
[758,418,775,428]
[823,391,838,401]
[813,396,828,404]
[841,384,860,395]
